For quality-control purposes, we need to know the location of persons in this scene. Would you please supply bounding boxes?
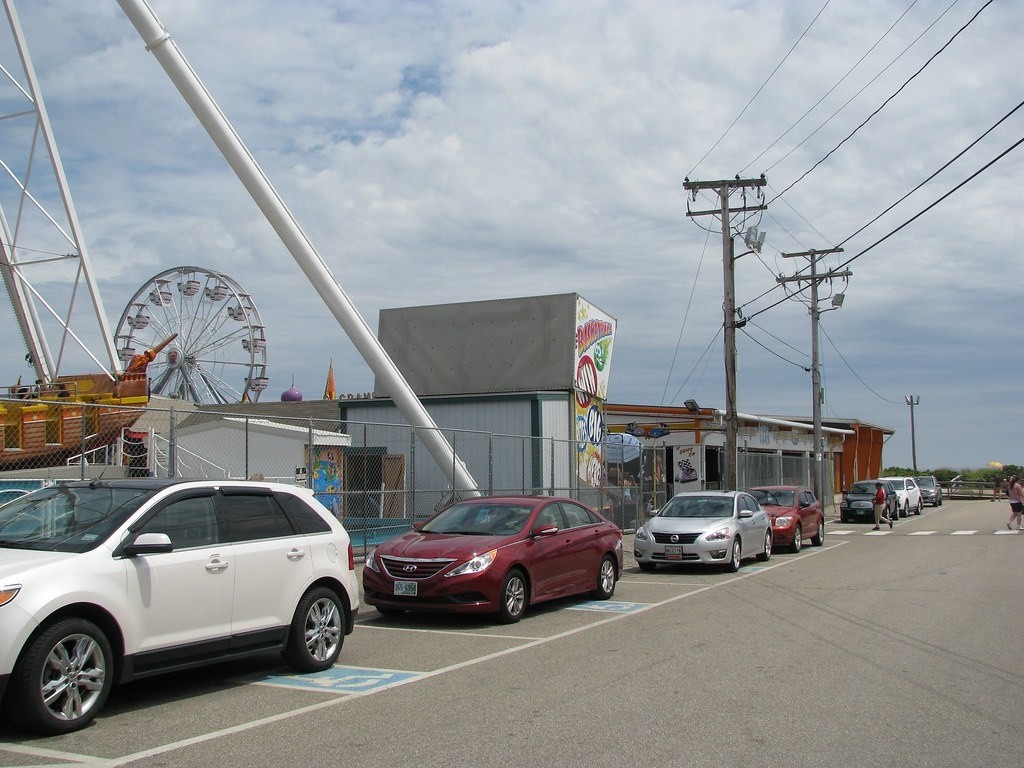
[871,482,893,530]
[11,385,38,399]
[608,464,638,500]
[1007,472,1024,530]
[991,474,1001,502]
[58,384,70,397]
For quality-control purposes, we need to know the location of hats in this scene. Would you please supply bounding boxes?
[1003,479,1006,480]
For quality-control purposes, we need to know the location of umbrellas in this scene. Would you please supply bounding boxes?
[607,433,643,486]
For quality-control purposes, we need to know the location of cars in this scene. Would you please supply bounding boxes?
[744,486,825,552]
[634,491,773,572]
[362,496,624,622]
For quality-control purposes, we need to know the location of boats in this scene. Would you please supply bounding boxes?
[0,332,179,469]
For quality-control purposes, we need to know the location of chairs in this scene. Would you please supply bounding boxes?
[543,515,558,528]
[172,515,211,548]
[554,512,579,530]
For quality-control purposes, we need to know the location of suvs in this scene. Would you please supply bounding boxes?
[0,480,359,731]
[912,475,942,507]
[877,477,923,517]
[840,480,900,522]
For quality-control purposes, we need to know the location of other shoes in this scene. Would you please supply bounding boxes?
[1016,527,1024,530]
[1007,523,1013,530]
[872,527,880,530]
[999,500,1000,502]
[889,522,893,529]
[991,500,995,502]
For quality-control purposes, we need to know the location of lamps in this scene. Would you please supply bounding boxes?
[683,399,700,413]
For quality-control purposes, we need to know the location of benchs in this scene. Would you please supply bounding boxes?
[70,393,114,415]
[0,401,42,424]
[34,397,81,420]
